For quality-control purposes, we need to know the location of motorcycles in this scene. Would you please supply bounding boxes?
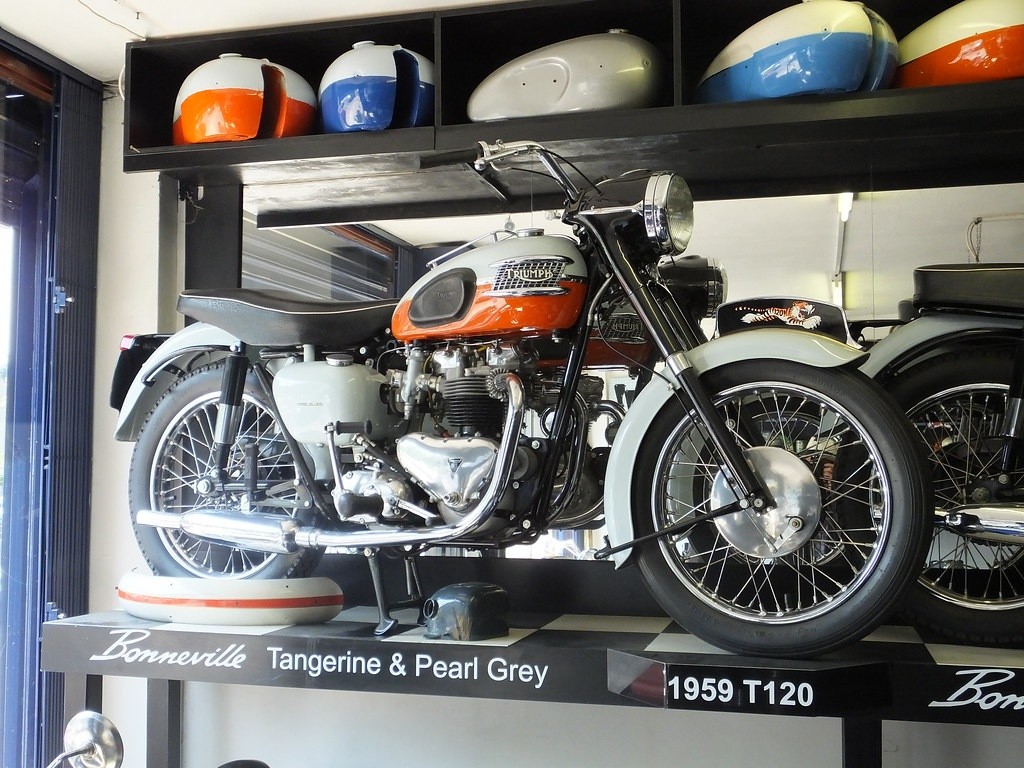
[830,263,1024,647]
[106,138,934,661]
